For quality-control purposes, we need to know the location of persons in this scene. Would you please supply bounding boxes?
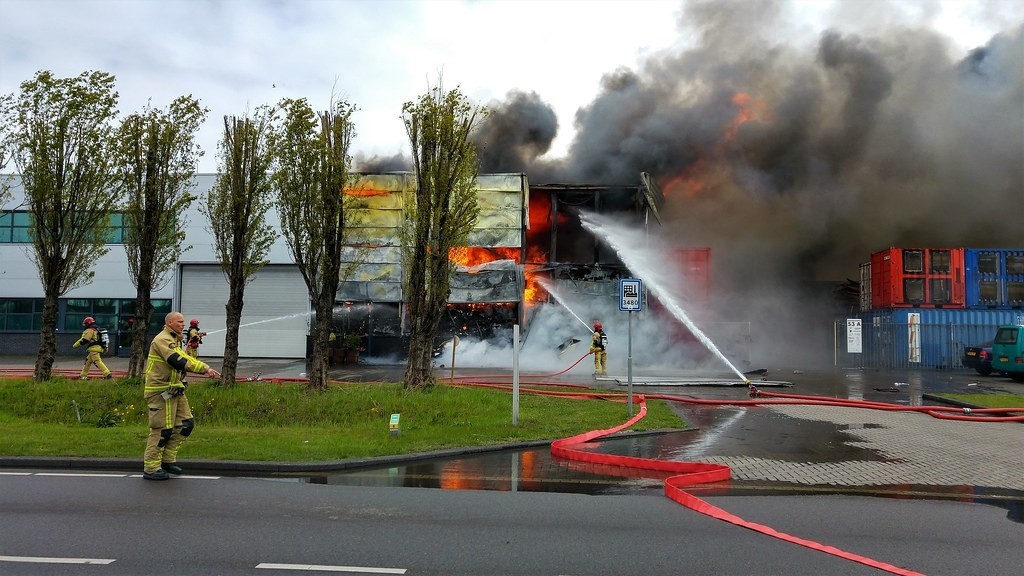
[590,322,608,376]
[143,311,221,480]
[185,319,203,359]
[72,317,111,380]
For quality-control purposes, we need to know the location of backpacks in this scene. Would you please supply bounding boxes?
[595,331,608,351]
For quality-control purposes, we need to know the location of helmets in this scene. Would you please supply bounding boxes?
[83,317,95,326]
[593,322,602,330]
[190,319,199,326]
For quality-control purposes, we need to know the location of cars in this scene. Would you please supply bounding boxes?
[961,340,1008,377]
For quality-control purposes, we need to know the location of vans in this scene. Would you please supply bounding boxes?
[990,324,1024,379]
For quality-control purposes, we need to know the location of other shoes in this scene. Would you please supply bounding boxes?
[161,463,183,474]
[79,377,87,380]
[103,374,112,380]
[143,469,170,481]
